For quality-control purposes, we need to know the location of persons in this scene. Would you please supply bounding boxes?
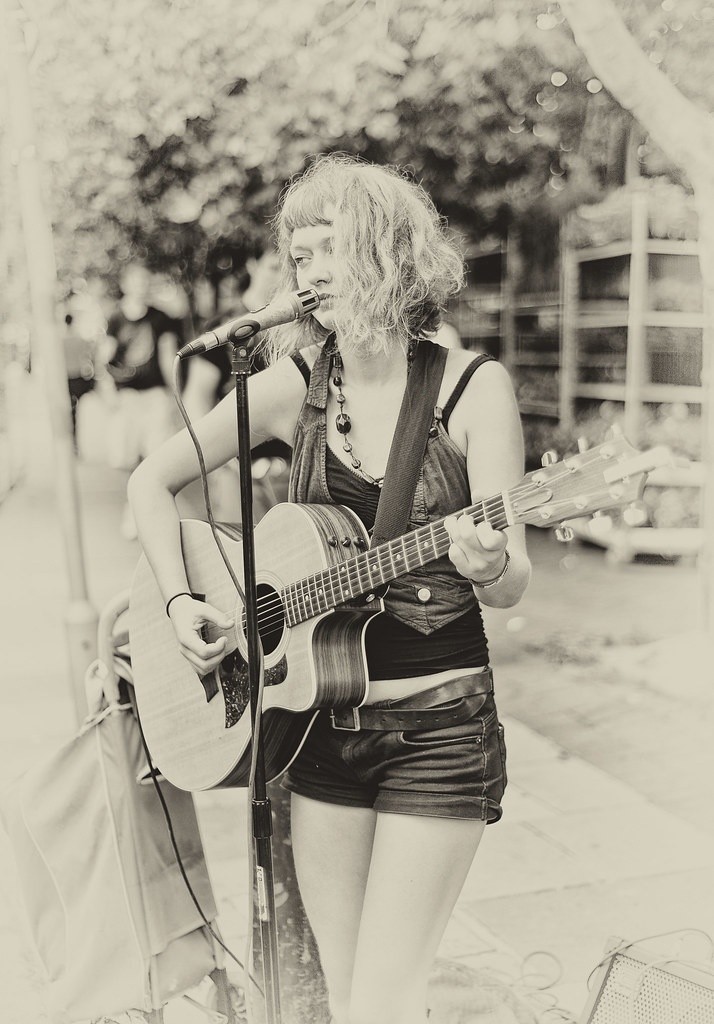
[125,156,530,1024]
[63,313,96,456]
[181,232,294,528]
[97,262,177,541]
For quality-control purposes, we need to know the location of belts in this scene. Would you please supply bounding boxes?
[322,669,491,730]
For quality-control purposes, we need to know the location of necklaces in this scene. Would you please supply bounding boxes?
[333,342,413,484]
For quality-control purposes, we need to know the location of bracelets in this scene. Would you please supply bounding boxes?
[166,593,193,618]
[468,549,510,588]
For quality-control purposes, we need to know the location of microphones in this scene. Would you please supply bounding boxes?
[176,289,320,360]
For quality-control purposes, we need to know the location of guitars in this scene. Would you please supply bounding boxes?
[128,423,658,791]
